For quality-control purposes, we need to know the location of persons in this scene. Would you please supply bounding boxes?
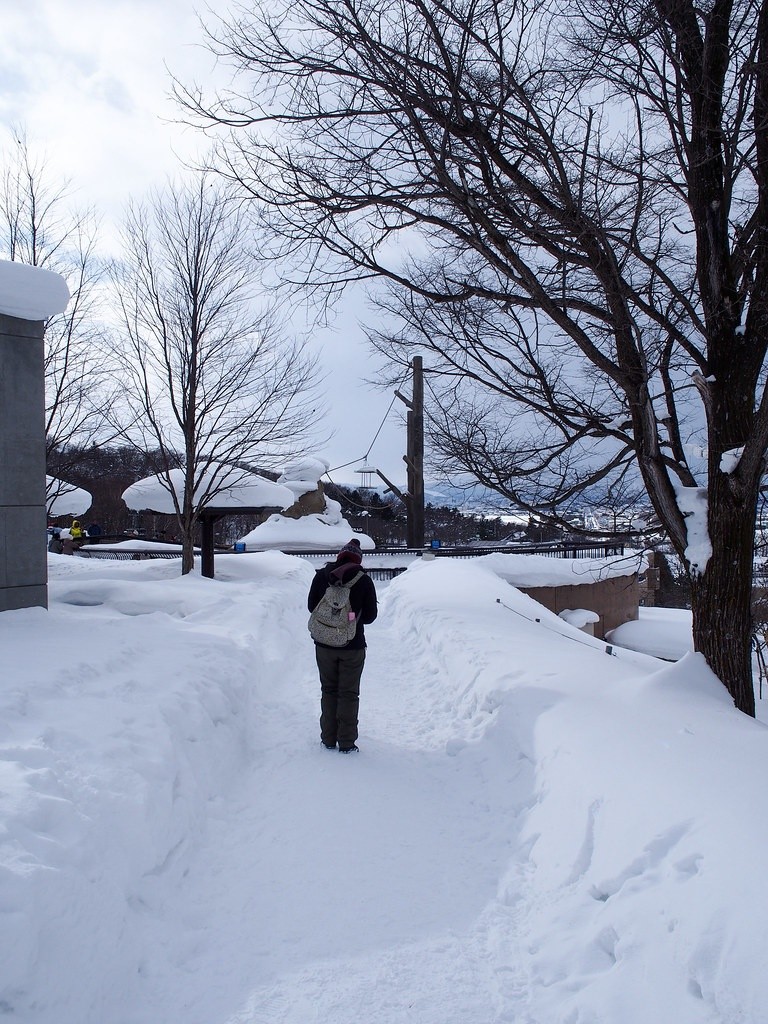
[70,519,84,545]
[61,538,79,555]
[171,534,178,542]
[48,523,62,538]
[88,519,102,544]
[307,538,377,754]
[48,533,64,554]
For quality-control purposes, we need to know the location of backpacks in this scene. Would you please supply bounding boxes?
[307,571,365,648]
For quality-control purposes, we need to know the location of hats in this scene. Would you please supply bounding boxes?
[53,533,60,539]
[337,539,363,564]
[65,534,73,541]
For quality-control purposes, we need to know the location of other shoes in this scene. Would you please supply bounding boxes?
[339,743,360,755]
[319,741,338,752]
